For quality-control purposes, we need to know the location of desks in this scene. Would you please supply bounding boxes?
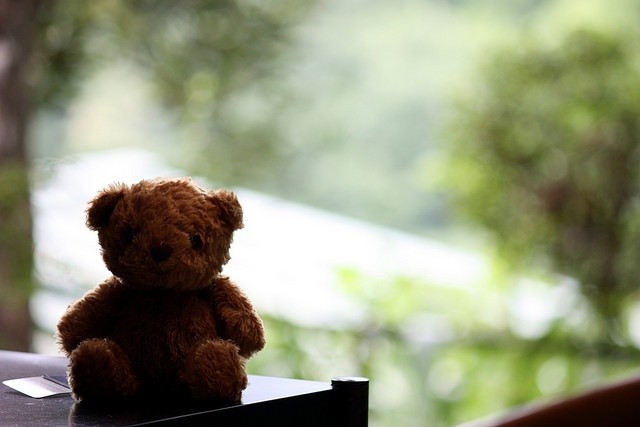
[1,350,370,427]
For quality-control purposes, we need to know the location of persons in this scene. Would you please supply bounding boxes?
[54,176,266,406]
